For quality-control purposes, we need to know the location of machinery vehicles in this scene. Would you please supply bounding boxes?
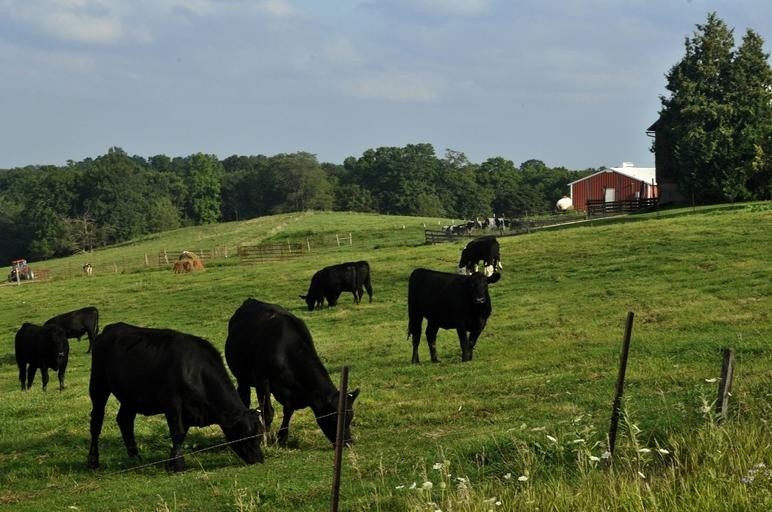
[8,259,35,282]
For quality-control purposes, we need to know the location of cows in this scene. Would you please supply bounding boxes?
[85,321,266,474]
[14,322,70,393]
[44,306,100,354]
[228,297,360,450]
[298,261,360,311]
[315,260,373,309]
[456,237,505,280]
[83,264,92,275]
[406,266,502,364]
[444,217,519,235]
